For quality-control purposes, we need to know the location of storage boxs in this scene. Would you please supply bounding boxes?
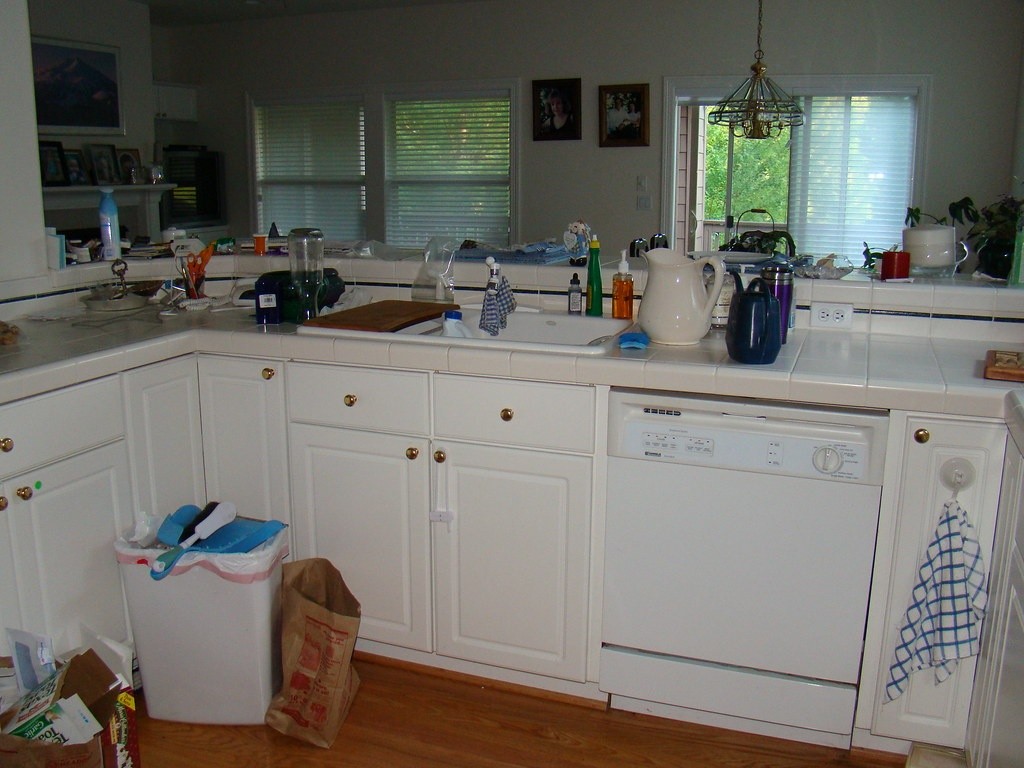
[0,648,140,768]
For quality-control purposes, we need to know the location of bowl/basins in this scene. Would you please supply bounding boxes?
[128,279,163,297]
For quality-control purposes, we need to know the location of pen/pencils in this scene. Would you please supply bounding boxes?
[180,245,214,299]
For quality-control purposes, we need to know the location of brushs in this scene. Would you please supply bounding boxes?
[149,501,236,581]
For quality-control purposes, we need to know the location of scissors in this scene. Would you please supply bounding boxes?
[187,253,202,277]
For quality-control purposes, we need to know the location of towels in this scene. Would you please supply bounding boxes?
[454,240,578,265]
[882,502,990,704]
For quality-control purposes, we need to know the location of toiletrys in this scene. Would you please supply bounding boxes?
[612,249,634,320]
[584,234,602,316]
[567,273,582,313]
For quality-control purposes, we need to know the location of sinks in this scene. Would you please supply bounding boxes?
[418,309,633,355]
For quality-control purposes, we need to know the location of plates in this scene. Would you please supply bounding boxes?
[790,258,854,279]
[688,250,777,262]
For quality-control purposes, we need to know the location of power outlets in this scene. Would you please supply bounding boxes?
[809,301,853,329]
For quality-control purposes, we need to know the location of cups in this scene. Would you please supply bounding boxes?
[650,233,669,249]
[758,266,795,345]
[130,161,148,184]
[629,238,649,257]
[252,233,269,254]
[881,251,910,280]
[149,161,168,184]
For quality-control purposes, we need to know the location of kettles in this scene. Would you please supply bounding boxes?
[731,209,774,253]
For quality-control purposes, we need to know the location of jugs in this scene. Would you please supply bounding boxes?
[724,270,783,365]
[638,248,728,345]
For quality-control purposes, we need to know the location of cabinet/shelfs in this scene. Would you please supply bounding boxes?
[0,352,207,663]
[153,81,199,123]
[852,412,1007,755]
[284,361,609,704]
[197,351,294,565]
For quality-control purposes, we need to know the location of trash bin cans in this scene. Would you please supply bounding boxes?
[114,516,289,724]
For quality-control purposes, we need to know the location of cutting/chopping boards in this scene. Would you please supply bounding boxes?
[302,299,461,333]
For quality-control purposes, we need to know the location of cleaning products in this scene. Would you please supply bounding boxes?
[438,311,472,338]
[97,187,122,260]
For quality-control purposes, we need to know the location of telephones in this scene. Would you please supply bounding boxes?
[233,278,258,306]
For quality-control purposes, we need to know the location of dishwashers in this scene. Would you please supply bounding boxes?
[604,388,895,753]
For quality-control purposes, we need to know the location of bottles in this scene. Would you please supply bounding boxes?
[568,273,582,315]
[99,189,123,261]
[586,235,604,317]
[287,228,327,321]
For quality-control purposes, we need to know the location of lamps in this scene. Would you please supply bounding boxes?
[708,0,807,139]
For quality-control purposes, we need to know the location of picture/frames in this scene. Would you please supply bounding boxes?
[38,140,72,188]
[532,78,582,141]
[599,83,650,147]
[116,149,142,185]
[63,149,94,186]
[30,34,127,138]
[81,143,123,186]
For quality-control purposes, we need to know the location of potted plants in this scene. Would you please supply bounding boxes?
[966,175,1024,279]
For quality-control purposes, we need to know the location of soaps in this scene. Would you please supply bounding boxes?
[619,333,649,349]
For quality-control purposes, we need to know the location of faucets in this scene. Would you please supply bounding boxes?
[484,255,501,295]
